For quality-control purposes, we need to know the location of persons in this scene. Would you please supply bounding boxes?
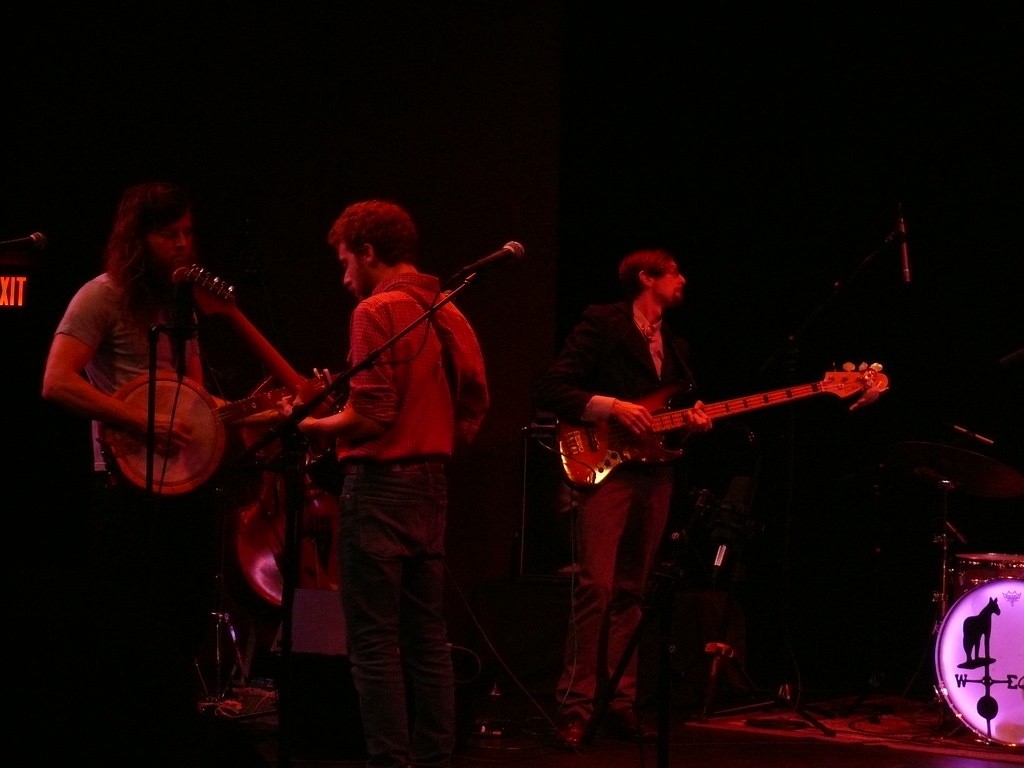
[40,183,302,768]
[278,198,491,768]
[539,249,714,751]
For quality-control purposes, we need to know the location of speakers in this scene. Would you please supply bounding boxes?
[279,587,368,761]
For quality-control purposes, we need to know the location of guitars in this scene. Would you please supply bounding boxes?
[106,365,356,493]
[171,263,355,457]
[559,360,890,494]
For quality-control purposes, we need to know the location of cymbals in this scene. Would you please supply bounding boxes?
[898,435,1024,501]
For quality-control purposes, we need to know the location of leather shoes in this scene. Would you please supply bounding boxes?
[553,710,588,746]
[613,705,659,737]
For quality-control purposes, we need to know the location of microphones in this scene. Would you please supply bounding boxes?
[896,214,912,283]
[458,240,525,277]
[950,424,994,447]
[0,231,46,254]
[170,266,194,378]
[1000,348,1024,364]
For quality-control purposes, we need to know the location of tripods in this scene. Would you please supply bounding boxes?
[706,630,838,738]
[191,488,249,702]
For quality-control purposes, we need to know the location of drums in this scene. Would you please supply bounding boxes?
[950,549,1024,600]
[933,574,1024,751]
